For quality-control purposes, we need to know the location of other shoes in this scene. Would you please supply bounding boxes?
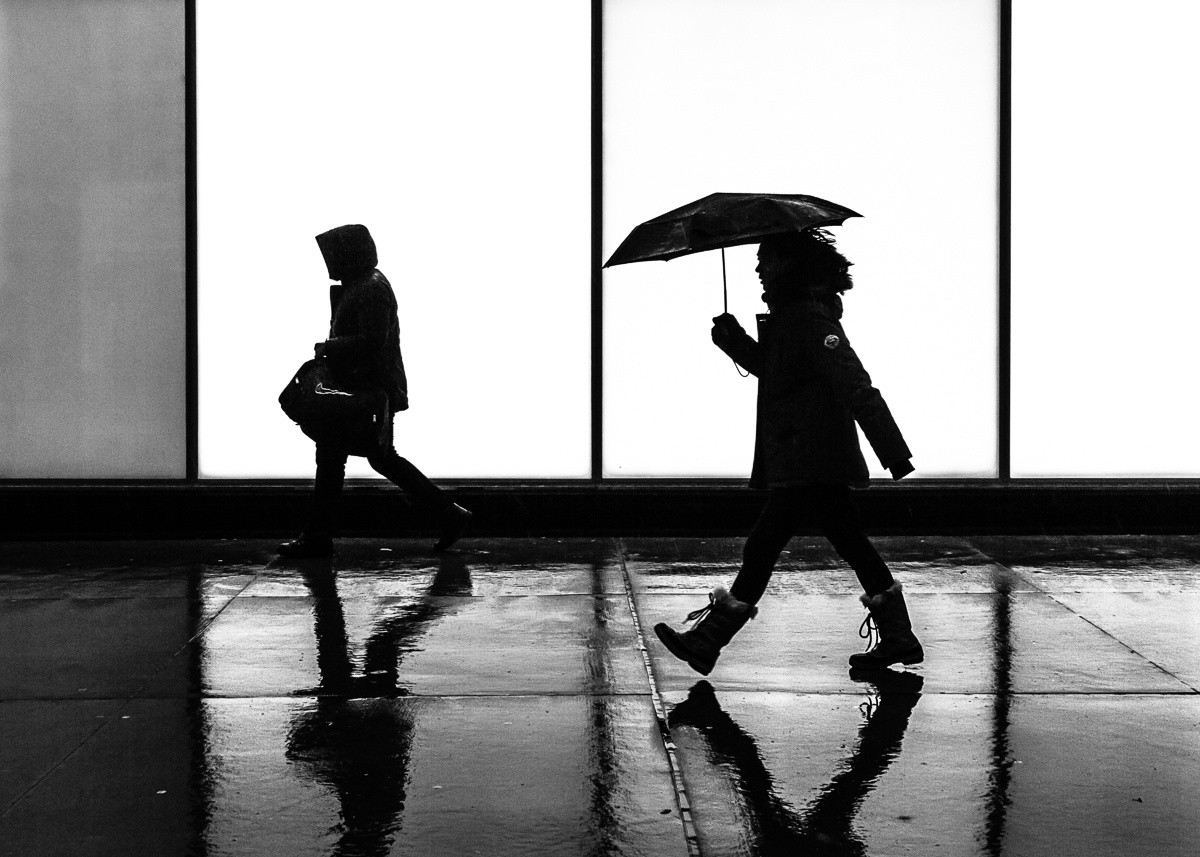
[279,531,335,556]
[433,501,475,550]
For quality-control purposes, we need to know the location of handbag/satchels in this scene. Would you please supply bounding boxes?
[277,345,392,454]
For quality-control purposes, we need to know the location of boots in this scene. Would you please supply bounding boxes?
[654,585,760,673]
[847,581,925,672]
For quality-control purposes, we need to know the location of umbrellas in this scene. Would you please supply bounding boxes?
[602,192,865,378]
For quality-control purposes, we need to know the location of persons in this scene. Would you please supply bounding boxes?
[654,231,924,677]
[274,225,473,557]
